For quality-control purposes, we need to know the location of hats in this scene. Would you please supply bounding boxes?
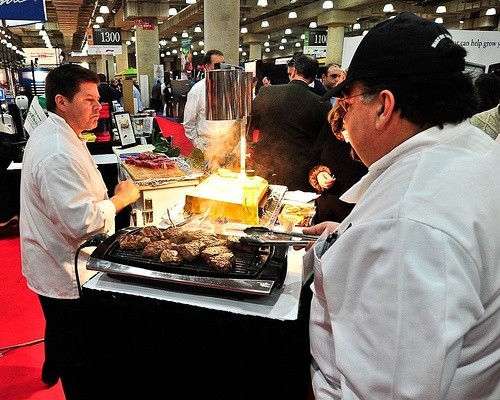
[319,11,465,102]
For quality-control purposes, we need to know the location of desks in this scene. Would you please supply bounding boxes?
[7,154,119,216]
[81,191,318,400]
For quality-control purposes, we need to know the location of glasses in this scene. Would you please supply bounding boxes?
[335,84,398,113]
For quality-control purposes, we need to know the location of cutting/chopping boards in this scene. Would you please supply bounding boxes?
[122,158,185,181]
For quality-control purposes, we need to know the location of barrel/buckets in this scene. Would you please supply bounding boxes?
[206,70,253,120]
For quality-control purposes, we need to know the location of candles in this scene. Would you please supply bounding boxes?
[240,137,245,168]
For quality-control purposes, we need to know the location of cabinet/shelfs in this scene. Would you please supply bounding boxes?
[112,144,199,225]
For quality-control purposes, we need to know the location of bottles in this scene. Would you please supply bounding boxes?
[242,169,260,226]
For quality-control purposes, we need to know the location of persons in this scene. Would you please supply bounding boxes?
[20,63,141,400]
[183,50,226,147]
[308,92,368,226]
[97,72,199,117]
[251,77,272,99]
[250,54,333,194]
[293,11,500,400]
[287,52,348,108]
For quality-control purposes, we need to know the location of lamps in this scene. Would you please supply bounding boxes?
[93,0,496,41]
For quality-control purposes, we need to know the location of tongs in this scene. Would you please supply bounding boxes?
[239,227,320,245]
